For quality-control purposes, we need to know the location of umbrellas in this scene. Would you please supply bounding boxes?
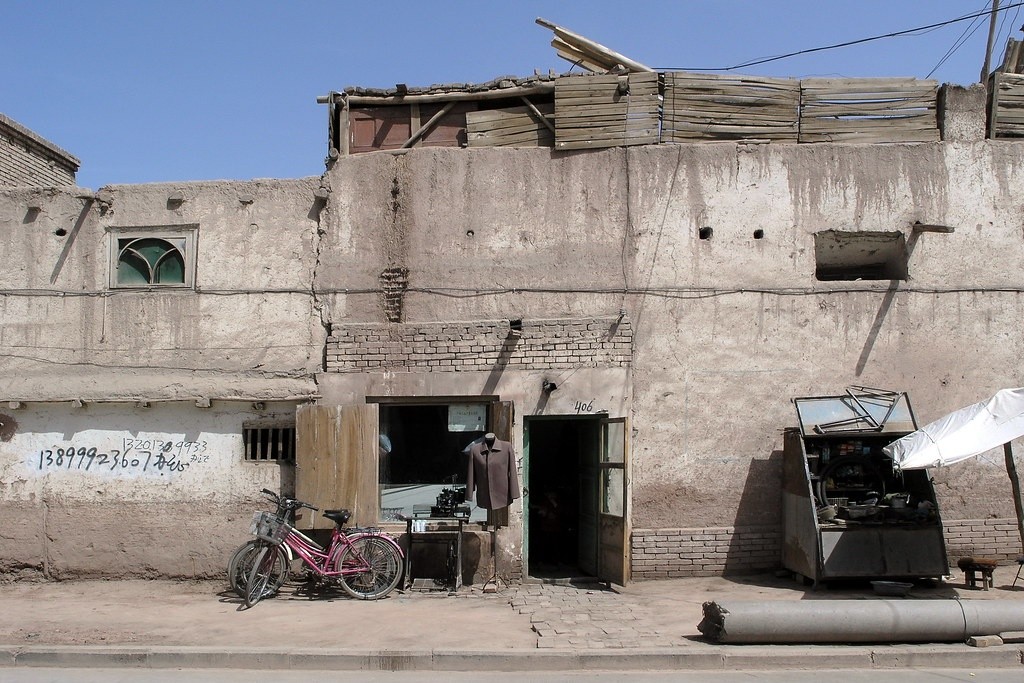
[883,386,1024,555]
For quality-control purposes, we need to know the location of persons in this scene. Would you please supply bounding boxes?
[466,433,520,510]
[530,488,579,568]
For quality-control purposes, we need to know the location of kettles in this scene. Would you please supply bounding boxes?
[890,493,910,509]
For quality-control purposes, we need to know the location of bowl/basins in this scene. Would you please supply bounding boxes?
[871,580,914,597]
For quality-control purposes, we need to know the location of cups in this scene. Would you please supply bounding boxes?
[411,520,427,532]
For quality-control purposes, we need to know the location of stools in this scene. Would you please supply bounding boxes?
[956,556,998,591]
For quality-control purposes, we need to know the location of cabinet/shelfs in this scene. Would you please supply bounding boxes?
[782,424,950,587]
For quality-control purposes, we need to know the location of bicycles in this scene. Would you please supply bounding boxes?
[226,487,406,608]
